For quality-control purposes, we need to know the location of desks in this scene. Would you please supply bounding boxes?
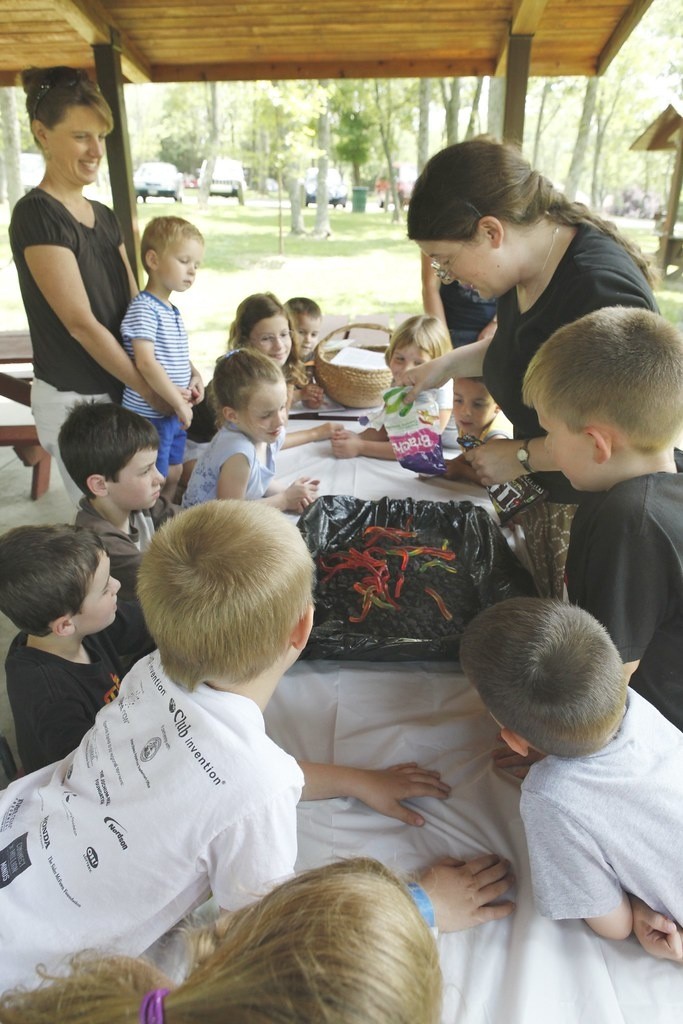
[146,310,683,1024]
[0,327,35,406]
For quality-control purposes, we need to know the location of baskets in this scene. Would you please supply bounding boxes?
[312,322,393,409]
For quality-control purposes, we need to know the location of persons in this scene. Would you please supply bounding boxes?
[326,247,514,494]
[460,597,683,962]
[393,141,662,611]
[61,402,166,665]
[522,305,683,728]
[120,217,204,504]
[10,65,201,508]
[181,349,321,515]
[0,856,445,1024]
[0,496,516,1000]
[0,523,148,777]
[183,291,325,489]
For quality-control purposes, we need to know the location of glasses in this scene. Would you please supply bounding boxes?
[431,215,482,282]
[249,329,295,346]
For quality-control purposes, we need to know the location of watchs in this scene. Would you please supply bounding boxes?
[516,439,537,474]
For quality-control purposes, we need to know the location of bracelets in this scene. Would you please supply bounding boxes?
[406,880,438,940]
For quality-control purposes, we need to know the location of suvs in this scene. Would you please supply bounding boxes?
[375,161,417,210]
[197,159,247,196]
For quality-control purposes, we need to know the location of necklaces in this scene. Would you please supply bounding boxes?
[536,226,561,282]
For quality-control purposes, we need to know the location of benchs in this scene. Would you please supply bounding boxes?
[0,422,60,499]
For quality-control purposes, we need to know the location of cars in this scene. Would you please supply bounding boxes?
[303,167,347,209]
[182,174,198,189]
[249,176,280,194]
[19,152,46,194]
[132,162,184,201]
